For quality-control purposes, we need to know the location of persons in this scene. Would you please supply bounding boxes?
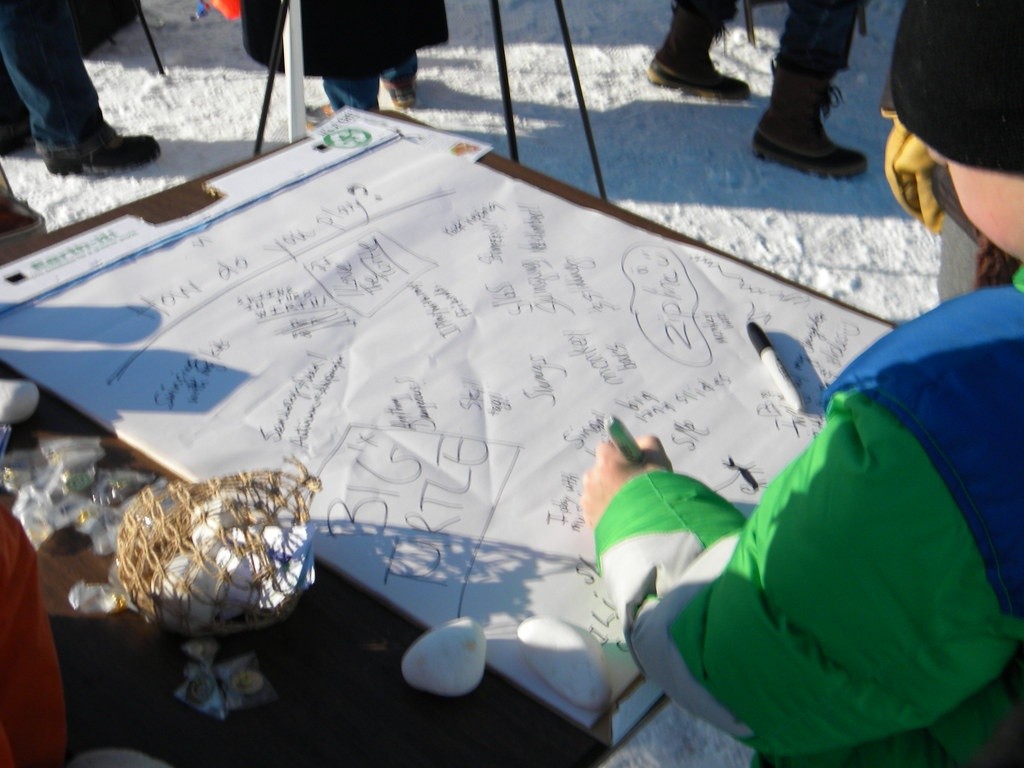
[584,2,1023,766]
[237,1,449,129]
[0,0,161,176]
[644,0,867,178]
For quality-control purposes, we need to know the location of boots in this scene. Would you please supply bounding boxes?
[752,66,870,177]
[646,8,751,100]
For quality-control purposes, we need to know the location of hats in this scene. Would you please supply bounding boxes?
[889,0,1024,176]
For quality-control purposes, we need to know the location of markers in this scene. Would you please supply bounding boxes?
[743,321,832,413]
[605,415,644,464]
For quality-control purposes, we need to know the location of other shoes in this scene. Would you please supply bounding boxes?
[305,104,379,130]
[0,118,30,156]
[41,134,160,174]
[383,79,416,110]
